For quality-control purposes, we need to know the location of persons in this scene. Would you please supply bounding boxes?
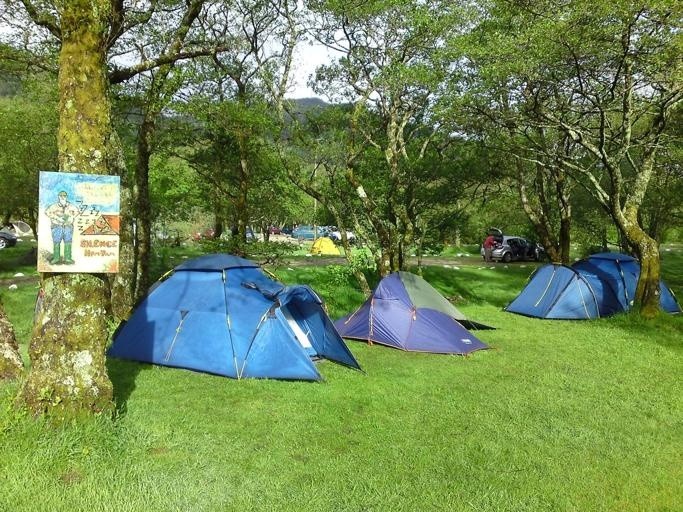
[483,234,497,262]
[45,191,80,265]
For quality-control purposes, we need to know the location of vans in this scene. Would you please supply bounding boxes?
[478,226,546,263]
[3,220,37,242]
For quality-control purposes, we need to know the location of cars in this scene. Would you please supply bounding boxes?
[193,222,357,246]
[0,231,19,250]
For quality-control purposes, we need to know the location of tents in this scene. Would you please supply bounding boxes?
[333,270,497,355]
[311,237,340,255]
[107,255,367,382]
[502,252,683,320]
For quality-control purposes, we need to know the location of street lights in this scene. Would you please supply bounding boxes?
[160,218,166,247]
